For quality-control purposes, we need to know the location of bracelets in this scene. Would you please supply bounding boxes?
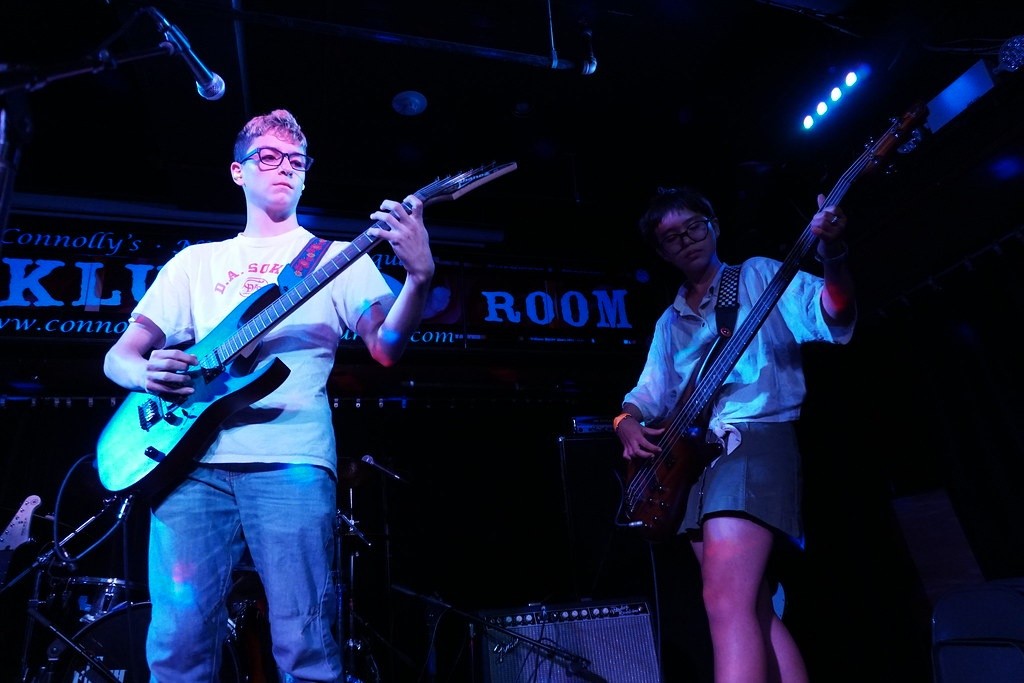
[814,249,850,265]
[613,413,633,432]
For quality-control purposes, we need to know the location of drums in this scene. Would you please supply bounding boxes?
[43,576,141,662]
[51,597,250,683]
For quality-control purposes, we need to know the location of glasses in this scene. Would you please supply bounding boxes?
[238,147,314,173]
[660,216,711,256]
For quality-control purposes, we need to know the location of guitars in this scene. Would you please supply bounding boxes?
[94,156,524,505]
[0,492,45,589]
[619,102,935,545]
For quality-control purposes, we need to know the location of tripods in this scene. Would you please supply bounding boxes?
[338,483,382,683]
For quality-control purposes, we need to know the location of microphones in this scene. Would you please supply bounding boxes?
[569,660,592,672]
[359,455,401,477]
[148,6,226,101]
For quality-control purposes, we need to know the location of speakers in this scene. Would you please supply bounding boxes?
[478,597,665,683]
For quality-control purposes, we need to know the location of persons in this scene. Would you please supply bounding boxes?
[613,190,852,683]
[103,110,435,683]
